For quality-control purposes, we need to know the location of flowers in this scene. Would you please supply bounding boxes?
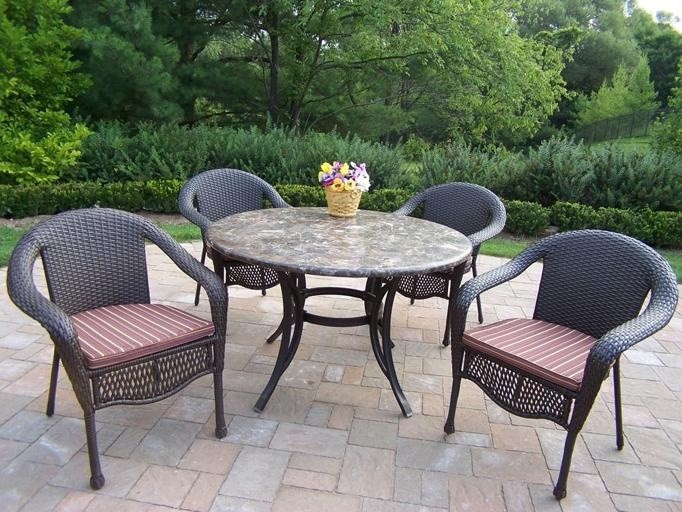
[318,159,371,193]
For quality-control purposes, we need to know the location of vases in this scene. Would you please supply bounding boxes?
[324,189,362,218]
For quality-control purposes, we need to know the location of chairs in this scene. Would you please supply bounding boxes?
[8,208,228,491]
[178,167,295,305]
[388,181,506,345]
[443,229,680,501]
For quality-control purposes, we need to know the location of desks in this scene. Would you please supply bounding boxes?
[203,207,473,419]
